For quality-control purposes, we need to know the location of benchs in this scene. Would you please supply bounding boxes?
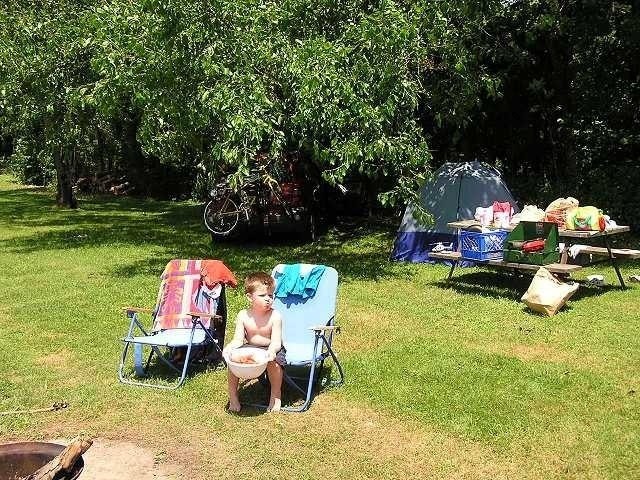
[428,240,640,290]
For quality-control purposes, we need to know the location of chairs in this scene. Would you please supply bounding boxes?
[120,257,227,390]
[239,262,343,412]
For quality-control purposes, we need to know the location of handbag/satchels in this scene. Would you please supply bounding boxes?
[564,206,606,232]
[520,266,581,318]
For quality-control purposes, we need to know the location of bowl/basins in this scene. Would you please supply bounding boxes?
[224,347,269,380]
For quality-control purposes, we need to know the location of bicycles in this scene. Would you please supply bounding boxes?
[202,149,309,237]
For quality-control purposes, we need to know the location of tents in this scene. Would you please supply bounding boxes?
[389,160,521,268]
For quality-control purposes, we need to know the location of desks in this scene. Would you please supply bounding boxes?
[446,220,630,293]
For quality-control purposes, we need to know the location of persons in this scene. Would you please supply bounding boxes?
[221,272,286,412]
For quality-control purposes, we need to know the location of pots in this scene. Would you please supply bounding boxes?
[0,442,85,480]
